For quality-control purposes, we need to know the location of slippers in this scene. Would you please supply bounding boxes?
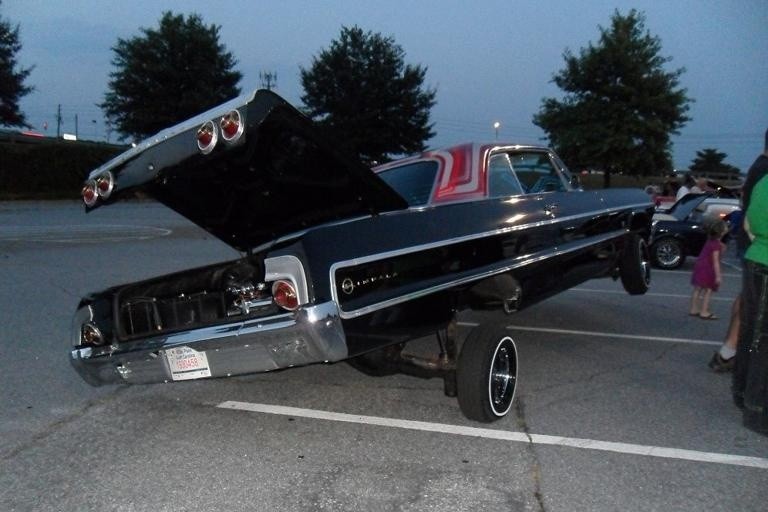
[688,311,718,320]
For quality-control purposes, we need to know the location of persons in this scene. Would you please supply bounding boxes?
[709,130,767,436]
[644,172,715,208]
[689,220,729,319]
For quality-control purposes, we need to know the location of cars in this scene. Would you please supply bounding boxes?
[647,176,746,271]
[69,89,658,422]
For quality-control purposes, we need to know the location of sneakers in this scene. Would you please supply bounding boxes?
[708,351,767,434]
